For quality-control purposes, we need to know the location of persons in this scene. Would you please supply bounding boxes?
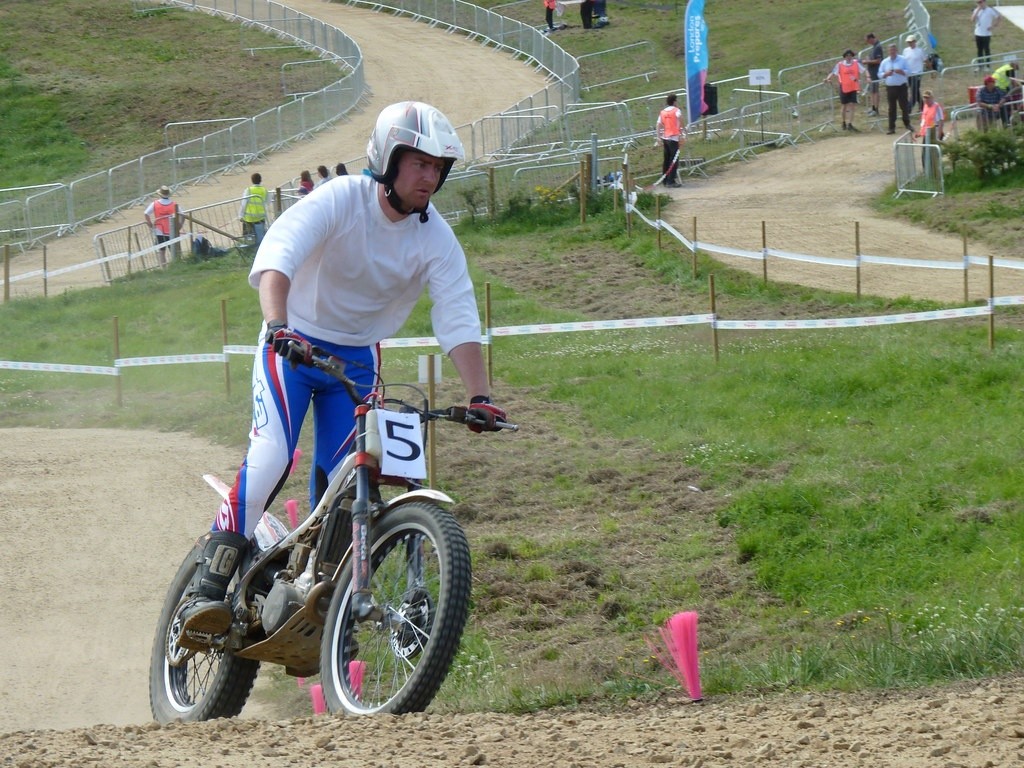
[144,185,184,267]
[238,173,270,243]
[177,101,507,679]
[298,170,314,196]
[902,35,932,112]
[824,49,872,133]
[914,90,946,173]
[580,0,609,29]
[335,163,348,177]
[976,61,1024,130]
[862,33,884,116]
[877,44,915,134]
[655,94,682,188]
[313,165,333,188]
[971,0,1001,71]
[544,0,555,31]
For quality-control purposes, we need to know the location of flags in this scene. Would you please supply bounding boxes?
[684,0,709,124]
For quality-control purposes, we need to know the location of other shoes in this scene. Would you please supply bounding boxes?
[886,130,896,135]
[665,182,682,188]
[863,108,873,114]
[842,122,846,130]
[974,67,983,73]
[867,111,879,117]
[984,67,992,72]
[906,125,915,130]
[847,125,855,130]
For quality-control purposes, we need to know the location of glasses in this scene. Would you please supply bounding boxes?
[977,1,984,4]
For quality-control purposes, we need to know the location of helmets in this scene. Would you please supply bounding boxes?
[365,101,466,195]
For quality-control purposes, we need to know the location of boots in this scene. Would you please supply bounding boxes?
[170,530,249,649]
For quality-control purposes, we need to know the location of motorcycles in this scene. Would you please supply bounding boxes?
[146,330,519,727]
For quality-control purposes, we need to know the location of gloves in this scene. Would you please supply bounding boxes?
[467,395,508,434]
[265,319,313,370]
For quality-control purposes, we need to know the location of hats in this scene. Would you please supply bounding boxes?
[843,49,855,59]
[156,185,172,198]
[905,35,916,42]
[984,75,997,82]
[921,90,934,97]
